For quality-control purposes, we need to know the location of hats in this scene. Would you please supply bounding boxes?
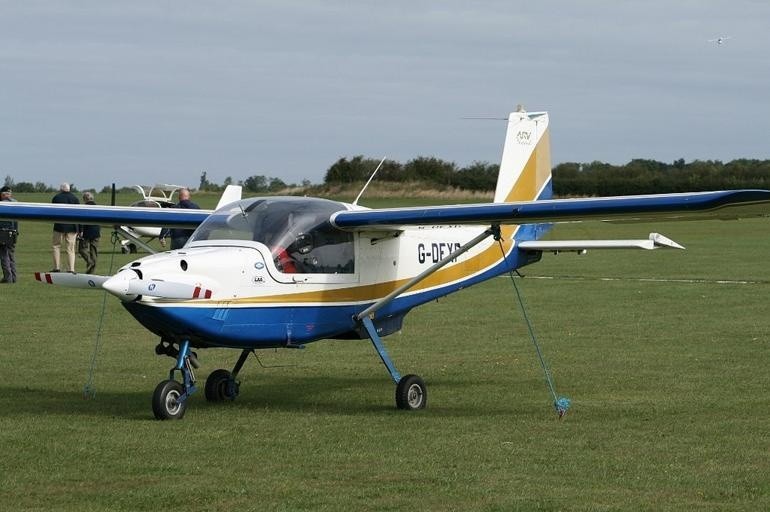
[0,186,13,193]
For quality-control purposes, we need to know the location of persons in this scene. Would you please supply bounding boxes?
[79,192,102,275]
[0,185,19,283]
[158,188,210,250]
[50,183,85,273]
